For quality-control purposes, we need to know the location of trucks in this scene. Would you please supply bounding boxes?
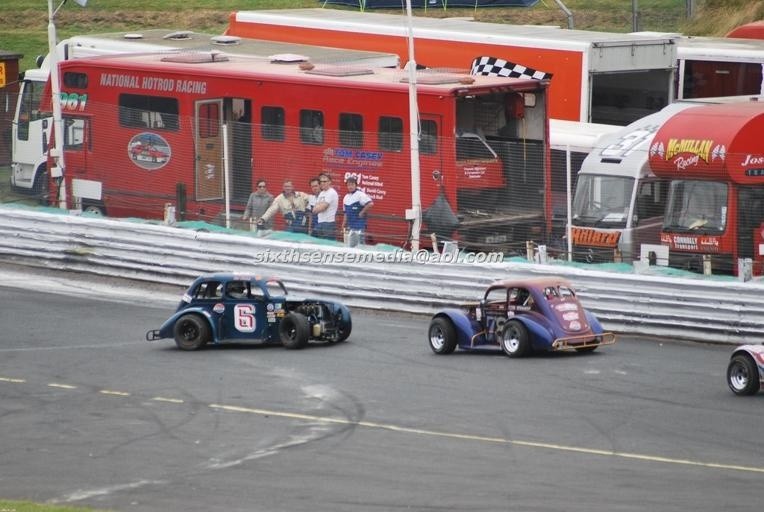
[12,26,400,206]
[222,8,678,127]
[723,21,763,40]
[40,51,552,262]
[563,94,764,265]
[648,102,764,278]
[627,28,763,103]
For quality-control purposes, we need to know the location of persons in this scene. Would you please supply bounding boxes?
[228,281,248,300]
[242,172,374,245]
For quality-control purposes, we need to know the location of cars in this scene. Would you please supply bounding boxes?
[149,271,354,349]
[423,129,503,212]
[429,276,617,356]
[726,339,764,396]
